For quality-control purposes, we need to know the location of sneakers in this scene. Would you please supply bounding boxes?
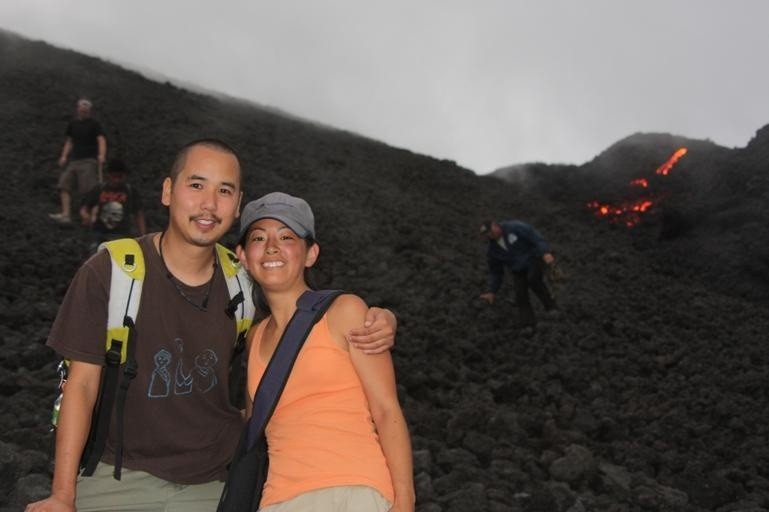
[48,212,70,226]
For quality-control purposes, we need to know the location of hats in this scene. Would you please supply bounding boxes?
[479,218,494,236]
[235,190,317,246]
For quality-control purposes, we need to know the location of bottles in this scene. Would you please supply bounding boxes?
[48,355,69,428]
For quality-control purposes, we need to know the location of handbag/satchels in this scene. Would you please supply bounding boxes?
[214,414,269,511]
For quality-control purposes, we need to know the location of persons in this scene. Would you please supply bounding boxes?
[230,192,418,512]
[48,98,107,224]
[481,217,560,337]
[59,159,147,253]
[23,135,397,512]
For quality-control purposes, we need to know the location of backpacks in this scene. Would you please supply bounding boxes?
[52,234,258,480]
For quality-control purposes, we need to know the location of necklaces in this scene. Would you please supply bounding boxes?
[159,227,218,309]
